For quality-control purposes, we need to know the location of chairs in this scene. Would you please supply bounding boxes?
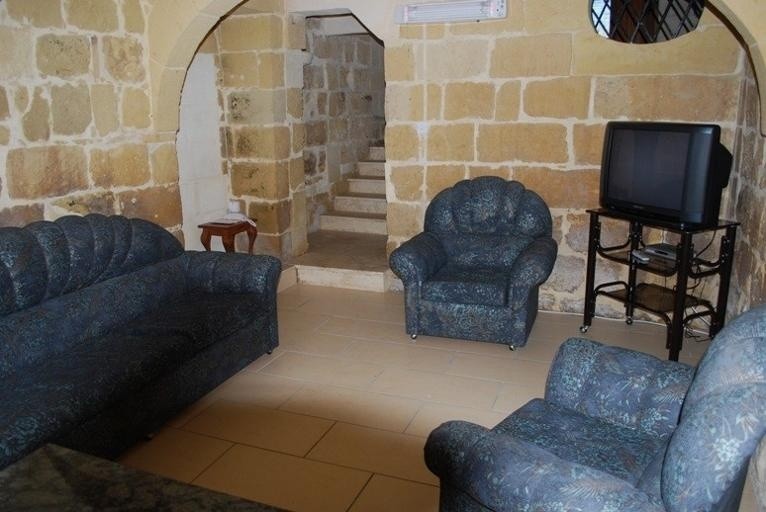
[424,305,764,512]
[387,176,557,347]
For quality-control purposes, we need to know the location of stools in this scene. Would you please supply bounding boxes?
[197,216,258,253]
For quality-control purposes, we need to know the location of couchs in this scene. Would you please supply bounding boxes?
[0,215,281,475]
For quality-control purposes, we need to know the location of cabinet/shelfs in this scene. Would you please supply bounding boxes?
[583,206,738,362]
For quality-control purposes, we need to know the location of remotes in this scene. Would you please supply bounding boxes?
[631,248,650,261]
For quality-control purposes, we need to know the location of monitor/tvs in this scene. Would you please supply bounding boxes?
[599,121,733,224]
